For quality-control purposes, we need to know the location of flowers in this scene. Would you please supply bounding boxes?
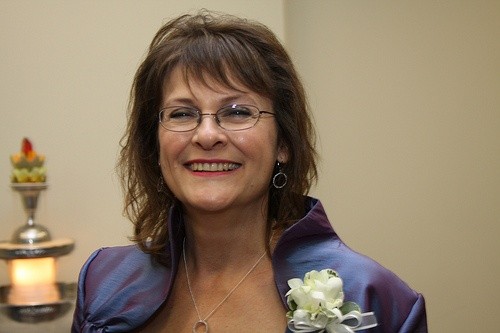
[285,269,377,333]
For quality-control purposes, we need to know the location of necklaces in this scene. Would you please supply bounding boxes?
[179,227,280,333]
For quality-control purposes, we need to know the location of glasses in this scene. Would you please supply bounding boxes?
[158,104,277,131]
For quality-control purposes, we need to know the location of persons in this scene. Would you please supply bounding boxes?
[67,8,428,333]
